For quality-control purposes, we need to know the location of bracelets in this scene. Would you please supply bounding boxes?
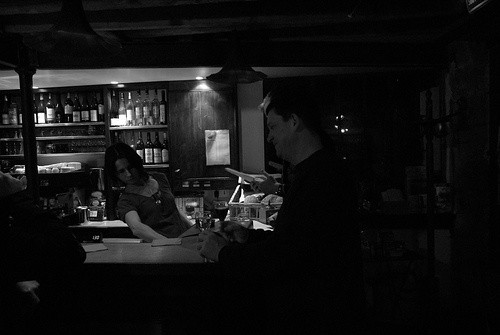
[273,180,283,196]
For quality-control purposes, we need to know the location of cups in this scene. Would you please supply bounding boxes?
[195,213,213,231]
[214,200,229,222]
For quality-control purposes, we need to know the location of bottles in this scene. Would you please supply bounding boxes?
[0,128,24,155]
[35,126,107,154]
[110,131,171,164]
[110,90,169,128]
[0,95,23,125]
[32,90,107,126]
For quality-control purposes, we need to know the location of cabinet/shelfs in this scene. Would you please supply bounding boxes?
[109,125,169,169]
[0,122,106,141]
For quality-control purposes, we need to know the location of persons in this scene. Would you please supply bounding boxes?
[104,142,193,242]
[0,230,40,335]
[0,171,87,335]
[244,170,283,199]
[198,84,362,335]
